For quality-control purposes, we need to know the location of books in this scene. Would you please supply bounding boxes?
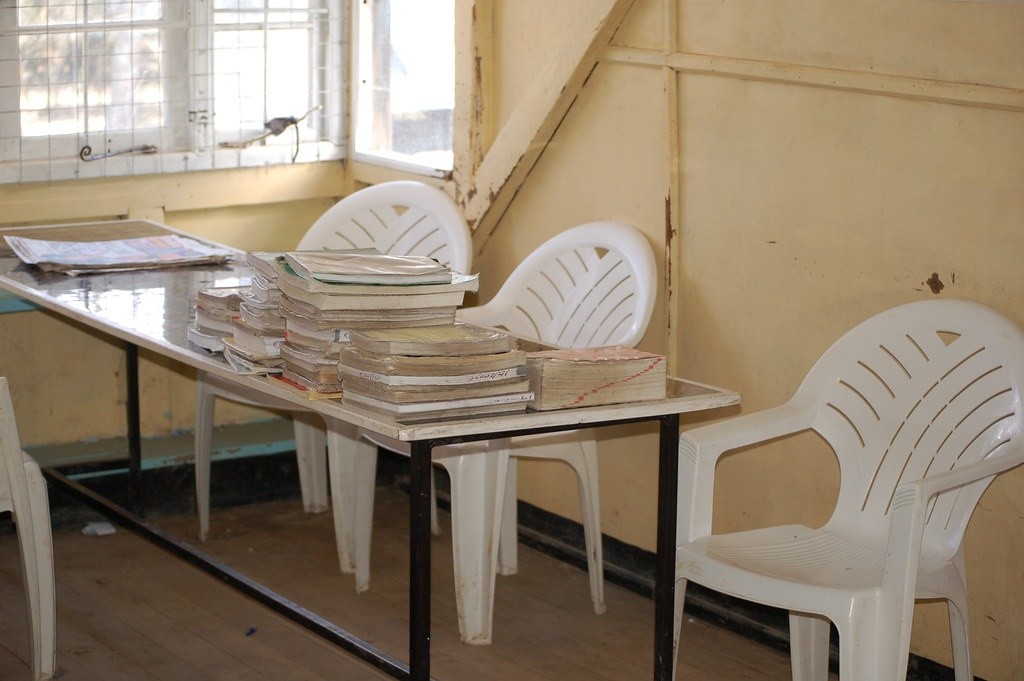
[187,247,666,422]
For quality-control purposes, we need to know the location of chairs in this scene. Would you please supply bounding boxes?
[351,223,661,645]
[193,179,473,573]
[670,295,1024,681]
[0,373,57,681]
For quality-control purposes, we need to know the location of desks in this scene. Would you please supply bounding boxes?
[0,220,742,681]
